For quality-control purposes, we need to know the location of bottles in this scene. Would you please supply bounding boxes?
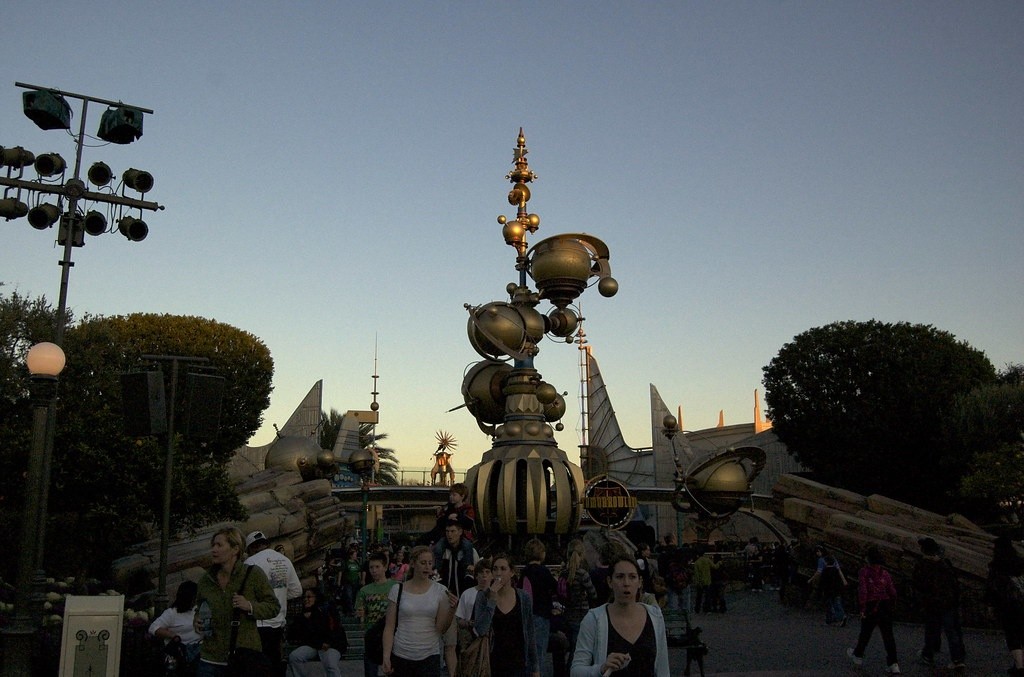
[198,598,212,637]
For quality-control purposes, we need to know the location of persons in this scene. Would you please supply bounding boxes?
[984,526,1024,677]
[807,546,848,626]
[286,588,348,677]
[637,536,787,618]
[243,531,303,677]
[570,552,670,677]
[383,546,460,677]
[148,580,204,663]
[430,484,478,595]
[192,526,283,677]
[324,544,409,677]
[912,537,967,669]
[847,547,900,674]
[456,540,597,677]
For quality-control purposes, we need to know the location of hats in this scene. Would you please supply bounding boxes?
[245,531,266,552]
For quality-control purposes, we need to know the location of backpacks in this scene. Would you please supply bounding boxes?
[657,548,681,589]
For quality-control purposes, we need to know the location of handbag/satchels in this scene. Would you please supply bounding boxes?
[548,589,573,617]
[652,575,668,592]
[226,647,287,677]
[364,615,393,665]
[453,631,491,677]
[160,636,189,673]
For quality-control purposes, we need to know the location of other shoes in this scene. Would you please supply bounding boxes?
[947,660,966,669]
[885,663,900,673]
[917,649,930,664]
[840,615,847,627]
[847,648,862,664]
